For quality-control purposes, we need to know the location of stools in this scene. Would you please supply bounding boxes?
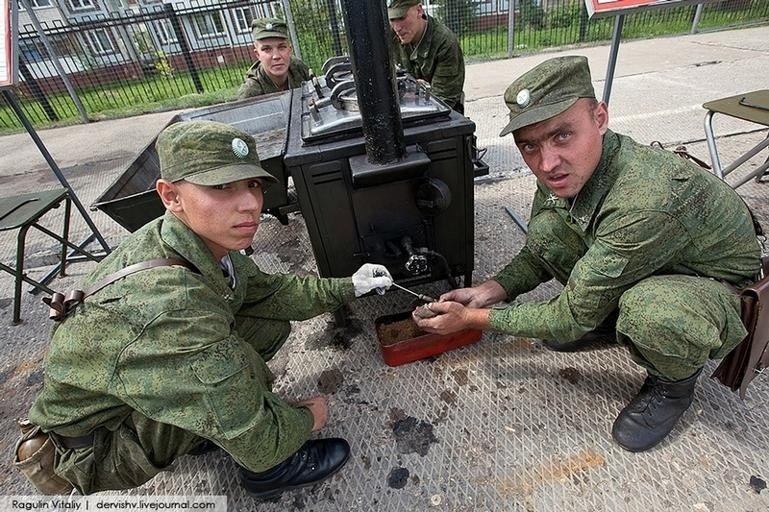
[703,88,768,190]
[0,186,102,326]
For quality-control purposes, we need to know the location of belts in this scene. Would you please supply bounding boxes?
[56,435,92,450]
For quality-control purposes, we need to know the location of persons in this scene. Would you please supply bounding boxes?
[24,117,394,496]
[385,1,466,118]
[233,14,317,103]
[410,57,760,452]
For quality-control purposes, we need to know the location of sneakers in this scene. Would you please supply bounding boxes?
[543,310,620,351]
[612,366,703,452]
[238,438,350,498]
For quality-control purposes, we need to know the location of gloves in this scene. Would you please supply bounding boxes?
[351,263,395,299]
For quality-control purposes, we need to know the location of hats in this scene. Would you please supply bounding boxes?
[153,120,280,187]
[251,17,288,41]
[497,55,596,137]
[387,0,420,20]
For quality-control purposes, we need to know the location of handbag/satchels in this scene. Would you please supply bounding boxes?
[712,278,769,403]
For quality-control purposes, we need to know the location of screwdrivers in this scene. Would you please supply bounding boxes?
[392,282,438,303]
